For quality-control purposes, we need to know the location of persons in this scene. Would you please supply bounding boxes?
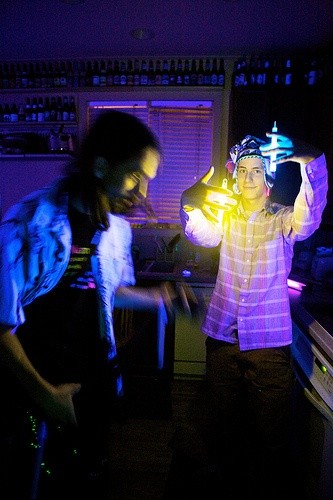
[0,108,194,500]
[180,132,329,424]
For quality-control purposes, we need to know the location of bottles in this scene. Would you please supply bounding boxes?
[0,95,76,122]
[0,57,226,90]
[231,52,333,86]
[48,123,73,151]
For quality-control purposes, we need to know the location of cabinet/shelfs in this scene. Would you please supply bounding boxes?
[174,282,215,381]
[0,54,333,160]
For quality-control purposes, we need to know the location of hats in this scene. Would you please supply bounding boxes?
[225,134,275,194]
